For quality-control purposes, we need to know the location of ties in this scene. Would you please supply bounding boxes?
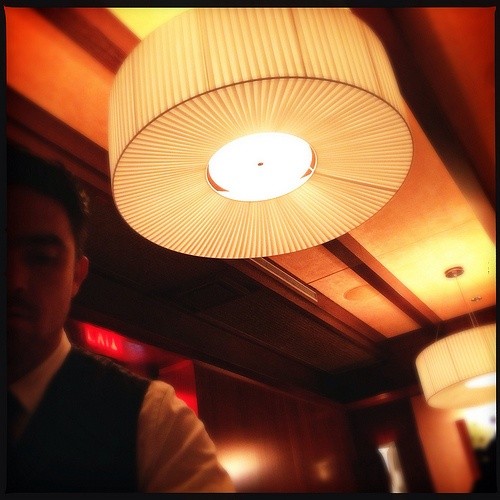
[5,390,27,490]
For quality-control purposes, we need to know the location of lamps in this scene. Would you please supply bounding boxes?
[107,7,412,259]
[415,265,496,411]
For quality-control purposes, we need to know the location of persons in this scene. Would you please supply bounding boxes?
[6,140,239,493]
